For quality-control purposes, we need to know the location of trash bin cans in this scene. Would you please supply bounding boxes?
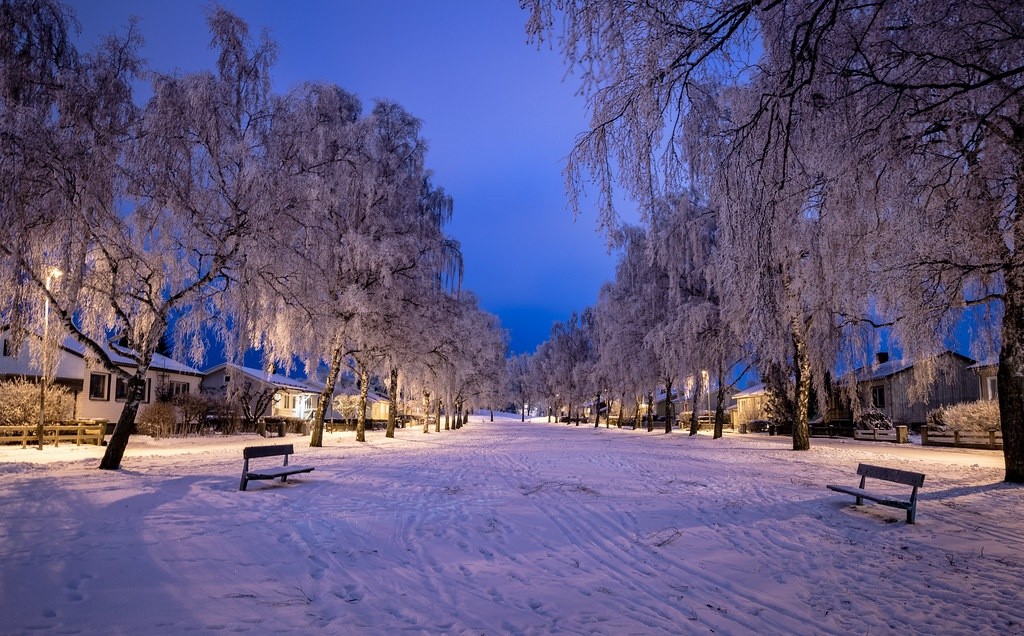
[77,417,110,446]
[257,418,267,438]
[278,422,288,436]
[302,421,311,435]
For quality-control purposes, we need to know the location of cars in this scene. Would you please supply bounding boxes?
[808,413,854,435]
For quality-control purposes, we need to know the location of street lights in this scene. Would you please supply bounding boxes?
[702,370,711,432]
[38,268,63,448]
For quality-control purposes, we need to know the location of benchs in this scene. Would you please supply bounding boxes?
[239,444,316,491]
[826,464,925,524]
